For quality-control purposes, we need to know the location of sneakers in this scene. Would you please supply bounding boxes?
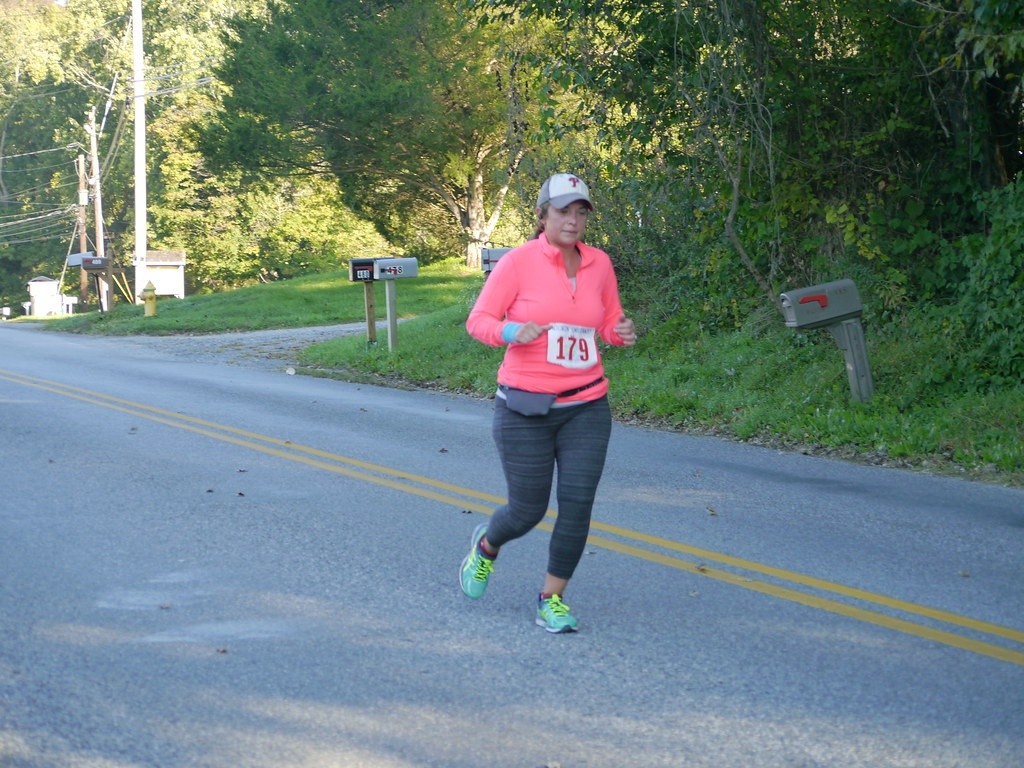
[536,592,578,633]
[459,523,500,600]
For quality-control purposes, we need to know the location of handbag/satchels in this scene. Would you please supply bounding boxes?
[499,378,603,417]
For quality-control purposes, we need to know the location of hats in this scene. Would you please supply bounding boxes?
[536,174,593,212]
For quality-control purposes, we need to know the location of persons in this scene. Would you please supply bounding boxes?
[459,172,637,634]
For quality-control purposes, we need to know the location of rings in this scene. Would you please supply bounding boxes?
[635,335,637,340]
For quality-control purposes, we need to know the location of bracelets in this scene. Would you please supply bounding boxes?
[502,321,523,343]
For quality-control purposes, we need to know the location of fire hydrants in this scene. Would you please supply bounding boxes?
[137,280,157,317]
